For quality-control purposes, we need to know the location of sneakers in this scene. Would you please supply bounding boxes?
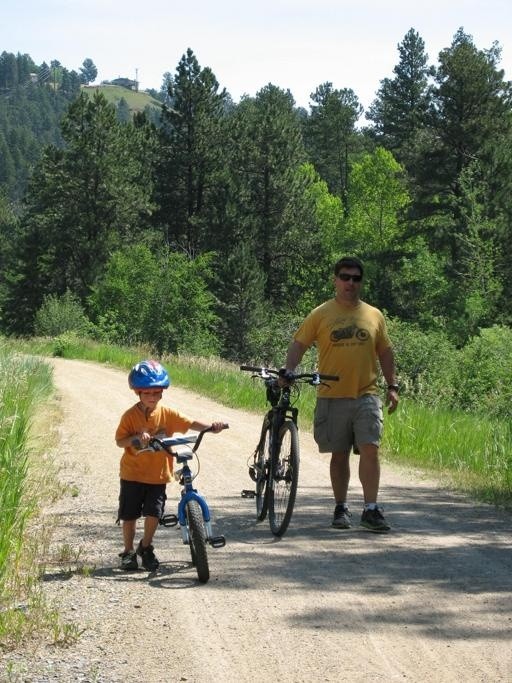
[119,549,138,569]
[136,539,159,571]
[332,502,353,529]
[360,504,391,530]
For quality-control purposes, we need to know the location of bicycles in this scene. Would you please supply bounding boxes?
[240,365,339,537]
[132,423,228,583]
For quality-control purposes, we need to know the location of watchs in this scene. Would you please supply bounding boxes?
[387,384,399,392]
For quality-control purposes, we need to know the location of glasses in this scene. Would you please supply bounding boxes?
[338,274,363,283]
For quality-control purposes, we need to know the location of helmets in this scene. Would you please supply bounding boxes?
[128,360,171,394]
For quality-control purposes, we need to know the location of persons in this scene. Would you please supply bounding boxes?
[113,358,225,570]
[277,254,401,533]
